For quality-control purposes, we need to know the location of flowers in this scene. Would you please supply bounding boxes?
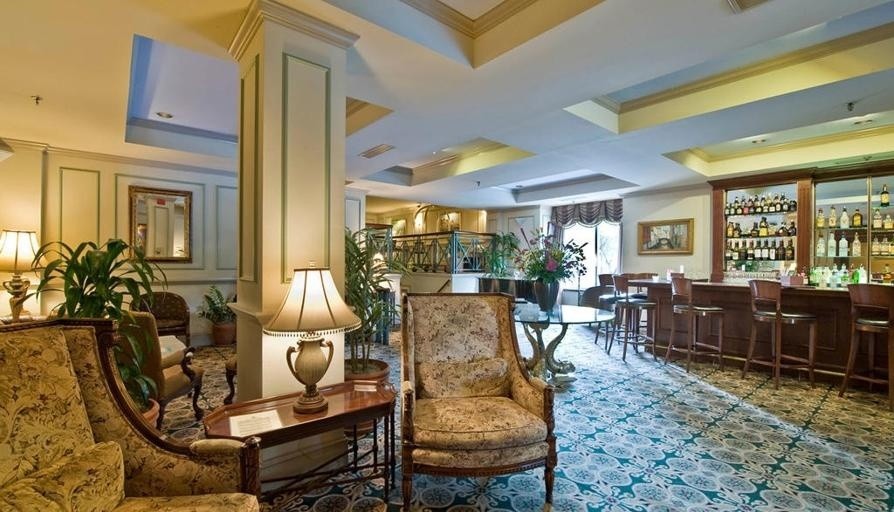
[513,224,589,285]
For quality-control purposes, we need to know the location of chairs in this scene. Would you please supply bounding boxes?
[399,290,558,512]
[581,285,616,326]
[0,316,263,512]
[128,292,191,348]
[112,304,206,430]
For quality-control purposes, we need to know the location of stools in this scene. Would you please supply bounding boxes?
[596,269,659,363]
[839,283,893,405]
[666,271,724,373]
[742,279,817,389]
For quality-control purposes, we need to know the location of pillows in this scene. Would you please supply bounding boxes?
[416,358,508,398]
[0,441,127,512]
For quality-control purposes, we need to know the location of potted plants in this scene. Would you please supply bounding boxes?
[341,221,400,436]
[30,241,168,430]
[195,284,237,345]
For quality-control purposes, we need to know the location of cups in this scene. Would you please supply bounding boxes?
[651,276,658,281]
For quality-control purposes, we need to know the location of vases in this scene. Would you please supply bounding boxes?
[533,276,559,313]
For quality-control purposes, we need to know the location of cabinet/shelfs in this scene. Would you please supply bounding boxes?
[707,158,894,284]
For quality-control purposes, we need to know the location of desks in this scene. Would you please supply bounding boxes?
[511,300,616,391]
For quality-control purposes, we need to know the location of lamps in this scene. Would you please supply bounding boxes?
[260,263,362,415]
[0,230,51,323]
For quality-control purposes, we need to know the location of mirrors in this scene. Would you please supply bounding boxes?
[128,184,192,263]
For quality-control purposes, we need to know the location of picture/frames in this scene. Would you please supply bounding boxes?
[637,217,694,255]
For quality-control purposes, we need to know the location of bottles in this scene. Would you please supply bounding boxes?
[724,185,894,287]
